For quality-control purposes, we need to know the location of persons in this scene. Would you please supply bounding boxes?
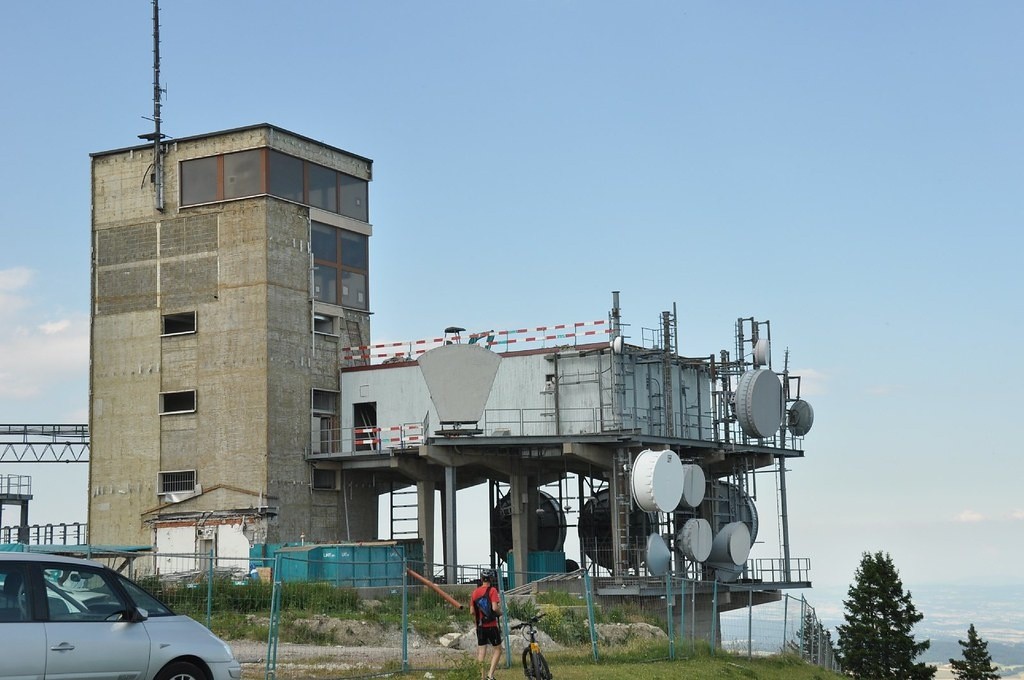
[470,570,502,680]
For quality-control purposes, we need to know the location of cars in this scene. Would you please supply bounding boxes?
[1,549,246,680]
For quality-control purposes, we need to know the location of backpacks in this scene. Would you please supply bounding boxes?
[474,586,496,628]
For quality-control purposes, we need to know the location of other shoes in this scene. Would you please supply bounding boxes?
[486,676,496,680]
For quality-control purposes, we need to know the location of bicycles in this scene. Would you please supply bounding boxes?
[510,613,558,680]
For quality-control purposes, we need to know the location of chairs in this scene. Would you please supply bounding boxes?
[0,574,28,622]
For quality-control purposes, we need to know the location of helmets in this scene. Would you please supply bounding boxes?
[480,568,494,581]
